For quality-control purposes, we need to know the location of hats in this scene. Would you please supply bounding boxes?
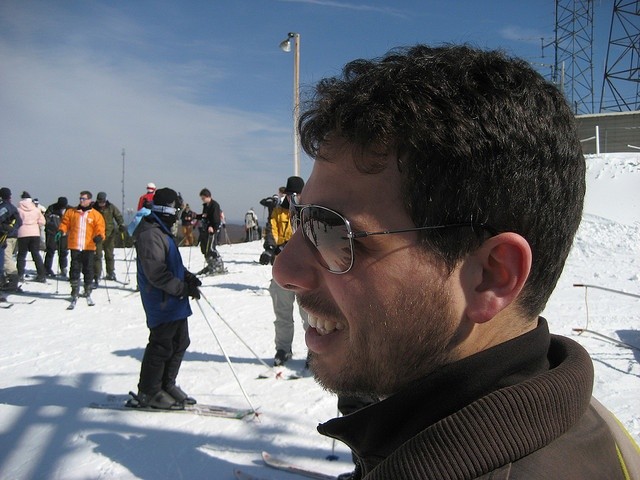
[153,188,179,216]
[21,191,31,198]
[283,176,304,193]
[0,188,11,201]
[58,197,67,209]
[32,199,39,203]
[97,192,106,203]
[147,183,156,192]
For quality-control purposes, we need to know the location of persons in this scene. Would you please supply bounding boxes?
[125,186,204,412]
[259,176,323,370]
[12,189,49,285]
[190,188,227,276]
[215,209,226,245]
[269,39,639,479]
[43,195,75,279]
[0,188,17,309]
[31,196,50,253]
[0,185,23,294]
[137,184,159,210]
[277,187,287,207]
[180,203,198,246]
[90,189,125,284]
[245,205,263,242]
[259,193,282,227]
[53,191,107,297]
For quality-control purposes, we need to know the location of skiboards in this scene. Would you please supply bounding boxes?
[93,277,129,289]
[0,299,36,308]
[87,394,262,420]
[67,296,95,310]
[259,365,309,378]
[195,267,243,278]
[234,451,337,480]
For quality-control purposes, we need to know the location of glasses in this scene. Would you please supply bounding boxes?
[80,198,88,201]
[289,192,497,274]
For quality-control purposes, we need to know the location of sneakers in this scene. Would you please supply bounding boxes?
[162,386,196,405]
[31,276,46,283]
[274,349,292,365]
[138,389,183,409]
[1,275,18,292]
[306,353,311,370]
[72,288,79,297]
[103,274,116,280]
[84,284,92,297]
[198,265,226,276]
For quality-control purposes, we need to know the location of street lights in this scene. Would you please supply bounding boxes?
[280,32,301,176]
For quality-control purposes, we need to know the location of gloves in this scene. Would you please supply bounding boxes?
[118,225,125,233]
[184,282,200,299]
[93,236,102,243]
[185,271,201,286]
[54,231,61,242]
[260,245,280,265]
